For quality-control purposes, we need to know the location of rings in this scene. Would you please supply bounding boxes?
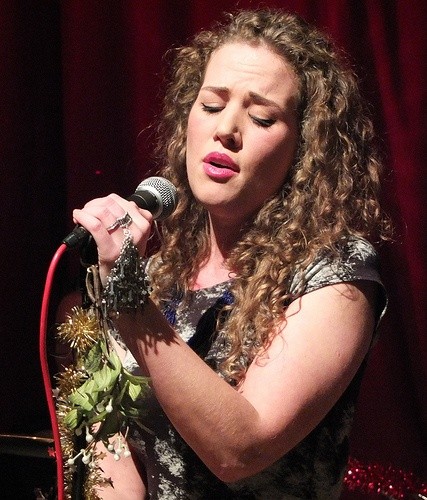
[106,222,119,231]
[117,212,132,228]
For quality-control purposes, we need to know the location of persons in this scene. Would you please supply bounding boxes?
[72,9,396,500]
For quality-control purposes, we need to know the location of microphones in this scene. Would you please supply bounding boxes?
[66,177,178,252]
